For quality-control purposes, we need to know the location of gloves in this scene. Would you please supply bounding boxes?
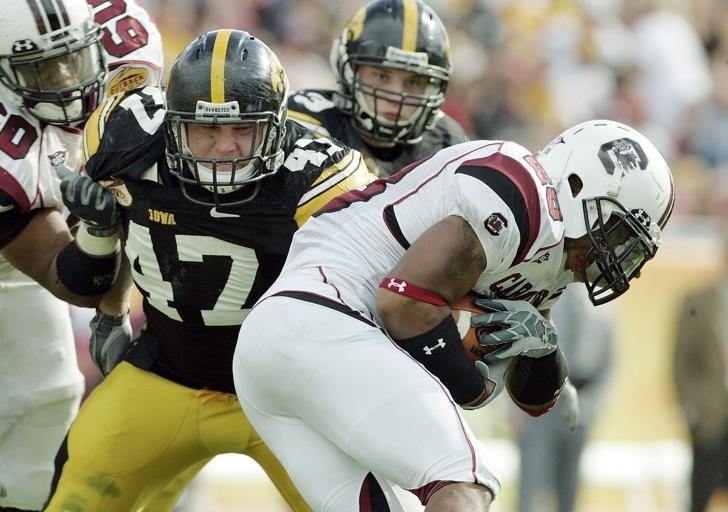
[55,164,118,228]
[461,298,558,416]
[561,379,579,433]
[88,305,134,379]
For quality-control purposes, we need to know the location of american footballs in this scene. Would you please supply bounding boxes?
[450,294,508,363]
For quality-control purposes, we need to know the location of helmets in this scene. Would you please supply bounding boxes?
[533,119,671,239]
[0,0,95,105]
[329,0,451,110]
[166,29,287,153]
